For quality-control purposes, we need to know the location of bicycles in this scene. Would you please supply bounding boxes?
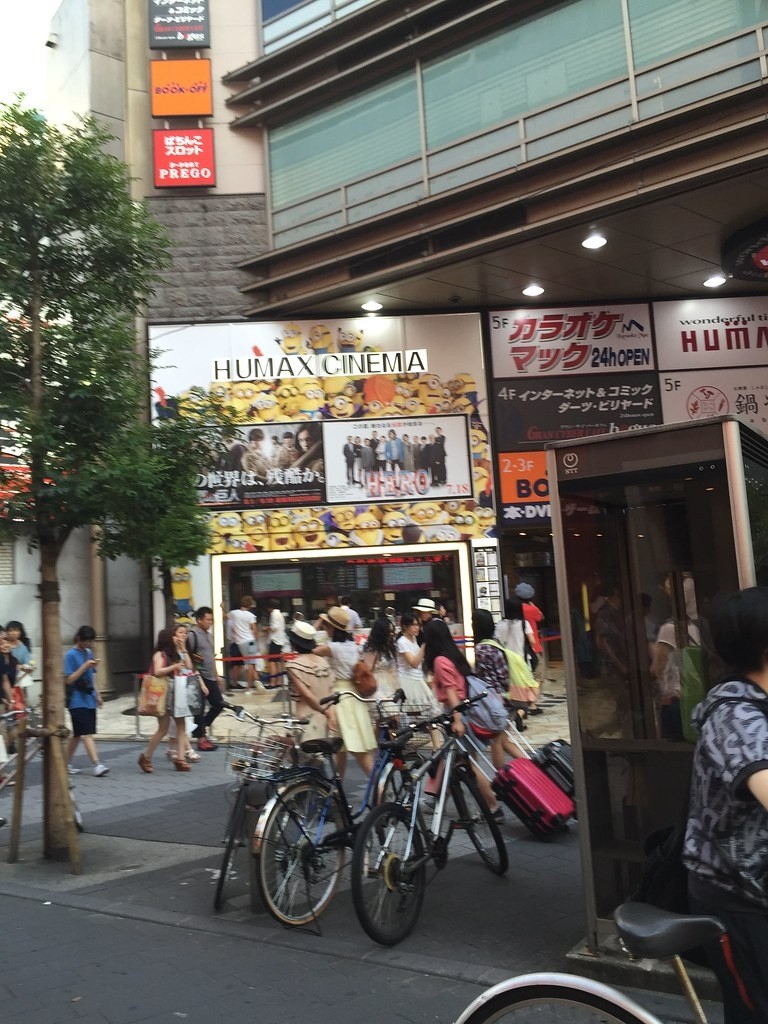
[212,698,314,914]
[250,689,441,937]
[0,692,87,836]
[351,692,509,947]
[451,903,731,1024]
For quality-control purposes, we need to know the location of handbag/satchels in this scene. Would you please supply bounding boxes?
[172,666,202,716]
[138,651,168,717]
[630,822,696,963]
[522,619,538,672]
[354,659,377,696]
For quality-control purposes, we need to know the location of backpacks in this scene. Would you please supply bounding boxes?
[476,637,539,707]
[463,671,508,737]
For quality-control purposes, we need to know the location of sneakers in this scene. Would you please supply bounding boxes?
[473,806,506,824]
[412,792,445,816]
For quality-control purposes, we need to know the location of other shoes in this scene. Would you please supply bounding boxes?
[0,776,16,786]
[62,739,217,776]
[230,681,288,696]
[528,707,544,714]
[515,710,528,731]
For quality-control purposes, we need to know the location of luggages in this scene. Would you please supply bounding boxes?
[452,730,579,842]
[503,718,574,801]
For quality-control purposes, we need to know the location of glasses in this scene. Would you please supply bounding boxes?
[410,623,420,627]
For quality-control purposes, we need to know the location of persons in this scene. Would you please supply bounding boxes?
[344,427,447,486]
[63,626,109,776]
[681,587,768,1024]
[218,424,324,476]
[220,583,546,821]
[138,607,224,772]
[591,563,703,741]
[0,621,36,786]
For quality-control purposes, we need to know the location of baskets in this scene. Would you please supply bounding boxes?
[1,718,36,754]
[225,728,290,780]
[368,703,433,749]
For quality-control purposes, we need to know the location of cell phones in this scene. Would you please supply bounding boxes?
[94,659,100,663]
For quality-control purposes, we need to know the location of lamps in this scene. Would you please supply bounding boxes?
[581,221,607,250]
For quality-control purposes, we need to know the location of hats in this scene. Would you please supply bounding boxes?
[319,607,351,631]
[412,599,439,615]
[241,596,252,606]
[514,582,535,599]
[284,621,317,650]
